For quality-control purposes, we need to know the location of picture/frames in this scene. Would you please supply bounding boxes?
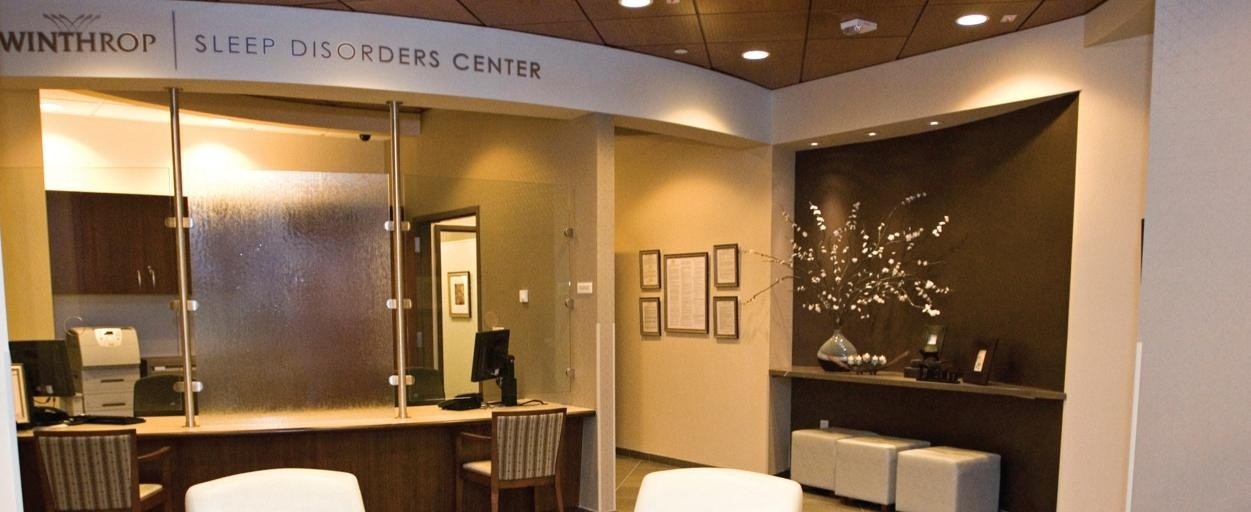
[713,295,739,339]
[446,270,473,319]
[663,252,709,335]
[638,249,661,289]
[12,361,31,427]
[712,243,739,287]
[638,297,662,337]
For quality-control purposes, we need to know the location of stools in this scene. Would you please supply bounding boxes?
[894,445,1001,512]
[835,434,931,506]
[790,427,878,493]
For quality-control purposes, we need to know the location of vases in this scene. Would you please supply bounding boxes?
[819,328,860,373]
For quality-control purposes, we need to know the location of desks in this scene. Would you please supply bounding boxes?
[19,397,597,446]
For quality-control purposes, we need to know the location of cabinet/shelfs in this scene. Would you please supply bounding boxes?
[65,324,142,419]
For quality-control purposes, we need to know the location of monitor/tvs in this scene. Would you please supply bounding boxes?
[470,329,511,382]
[8,340,75,397]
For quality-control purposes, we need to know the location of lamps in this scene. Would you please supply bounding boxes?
[955,15,990,29]
[840,18,879,37]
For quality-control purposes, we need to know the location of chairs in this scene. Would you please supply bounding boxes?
[31,429,172,511]
[457,407,567,512]
[132,373,186,418]
[634,468,805,511]
[395,367,446,407]
[184,469,370,512]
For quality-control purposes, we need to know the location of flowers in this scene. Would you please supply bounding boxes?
[740,194,952,327]
